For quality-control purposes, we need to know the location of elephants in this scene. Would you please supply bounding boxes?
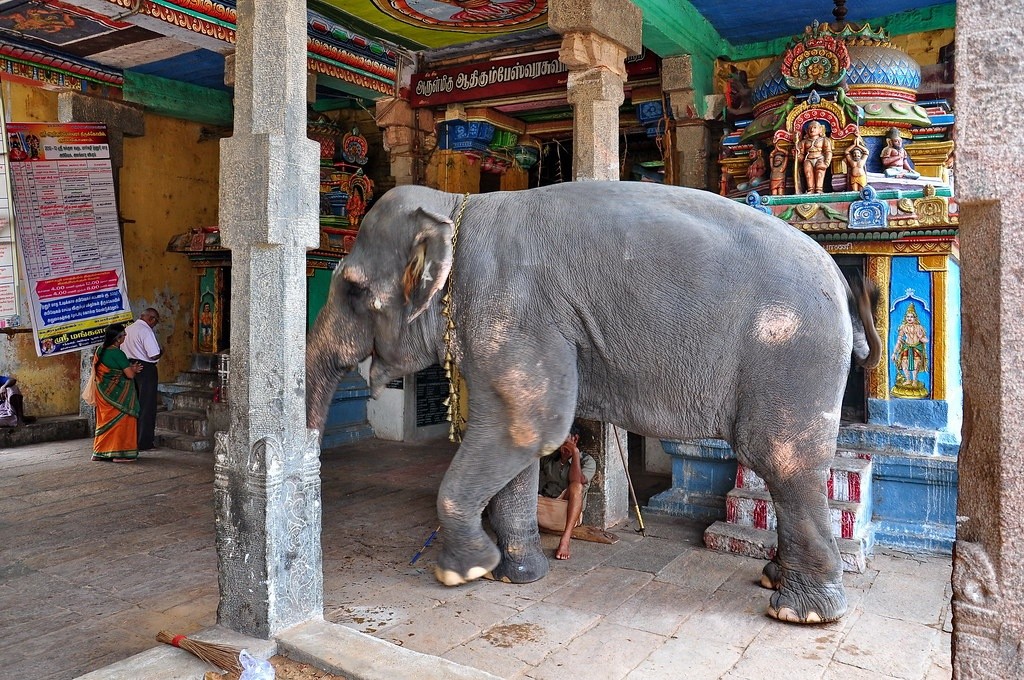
[305,180,881,626]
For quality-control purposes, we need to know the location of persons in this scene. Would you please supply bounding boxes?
[880,127,920,180]
[537,425,596,559]
[0,376,25,426]
[90,308,165,462]
[769,143,788,195]
[737,147,766,190]
[891,302,929,386]
[844,139,869,191]
[792,121,832,194]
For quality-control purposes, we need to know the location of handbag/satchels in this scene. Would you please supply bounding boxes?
[537,487,583,532]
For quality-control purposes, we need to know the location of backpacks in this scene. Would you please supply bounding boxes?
[0,397,18,427]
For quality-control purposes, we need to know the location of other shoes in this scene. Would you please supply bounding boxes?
[113,457,136,462]
[91,455,111,461]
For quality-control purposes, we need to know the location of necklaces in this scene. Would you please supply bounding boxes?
[561,462,565,466]
